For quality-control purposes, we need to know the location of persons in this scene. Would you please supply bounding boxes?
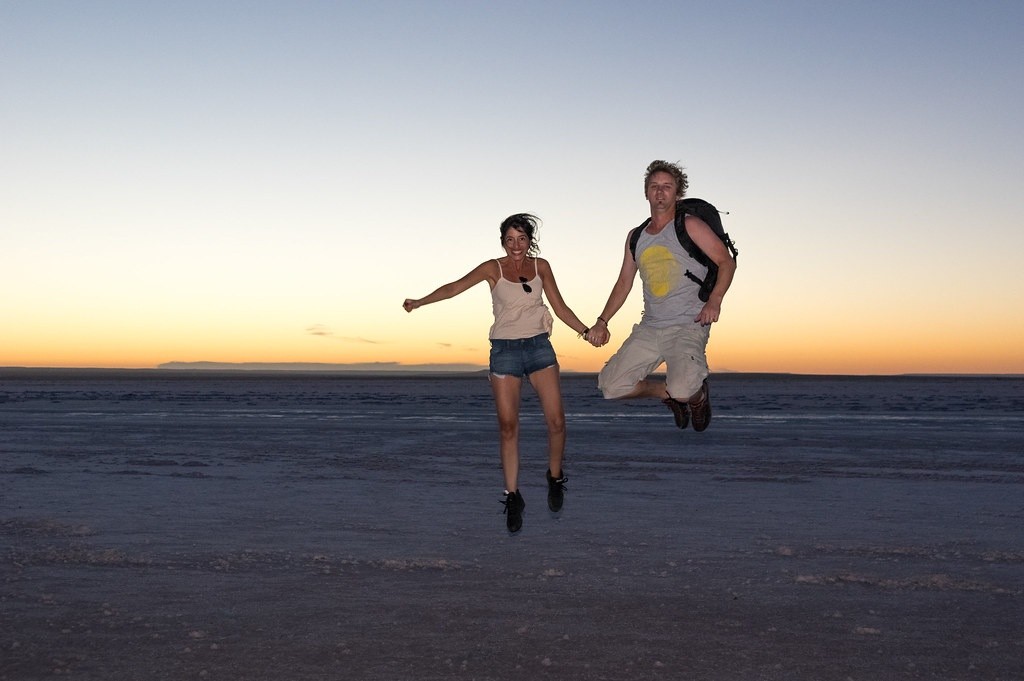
[586,161,737,432]
[402,213,607,533]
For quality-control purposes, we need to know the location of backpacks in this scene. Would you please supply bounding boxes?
[629,198,738,302]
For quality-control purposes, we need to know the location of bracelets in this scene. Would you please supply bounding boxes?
[577,326,589,339]
[597,315,608,328]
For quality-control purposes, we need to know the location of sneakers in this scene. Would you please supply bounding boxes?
[688,382,711,432]
[546,468,568,513]
[497,489,525,533]
[662,390,689,429]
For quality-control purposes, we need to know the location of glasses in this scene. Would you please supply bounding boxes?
[519,277,532,294]
